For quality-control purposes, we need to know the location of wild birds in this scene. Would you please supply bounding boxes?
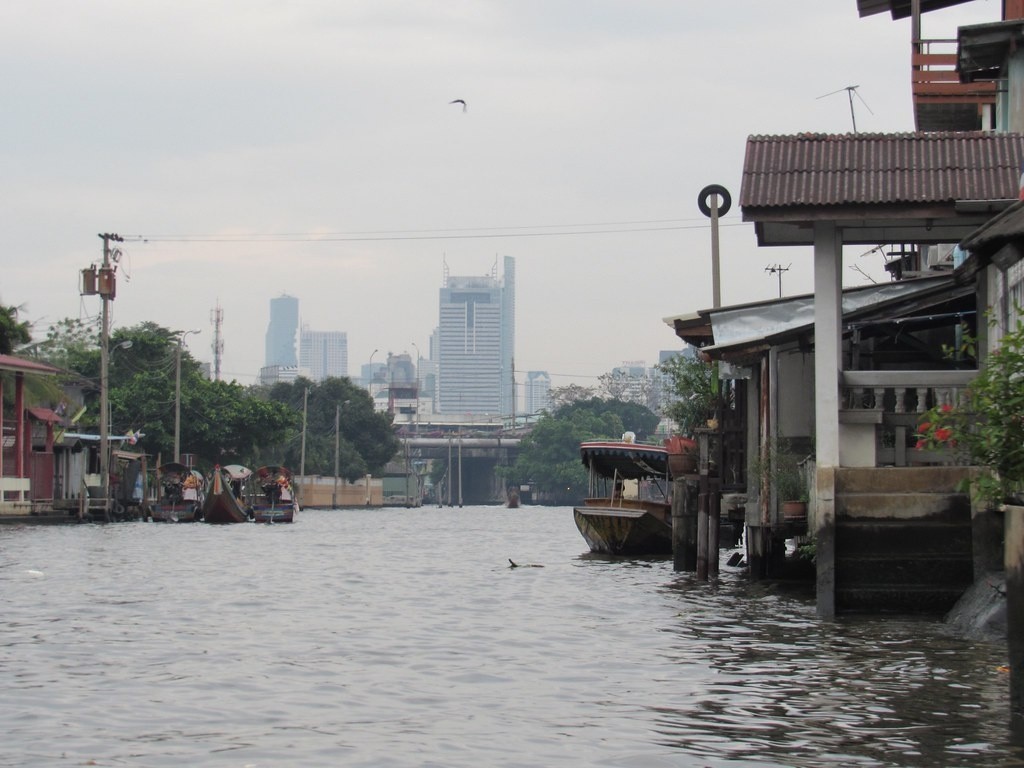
[449,100,468,112]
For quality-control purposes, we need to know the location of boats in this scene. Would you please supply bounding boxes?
[203,463,253,524]
[572,431,673,556]
[250,464,295,523]
[150,463,204,523]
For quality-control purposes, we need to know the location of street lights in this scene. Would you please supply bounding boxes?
[332,400,351,509]
[300,381,322,511]
[174,329,201,464]
[412,343,419,434]
[369,350,378,396]
[101,341,133,497]
